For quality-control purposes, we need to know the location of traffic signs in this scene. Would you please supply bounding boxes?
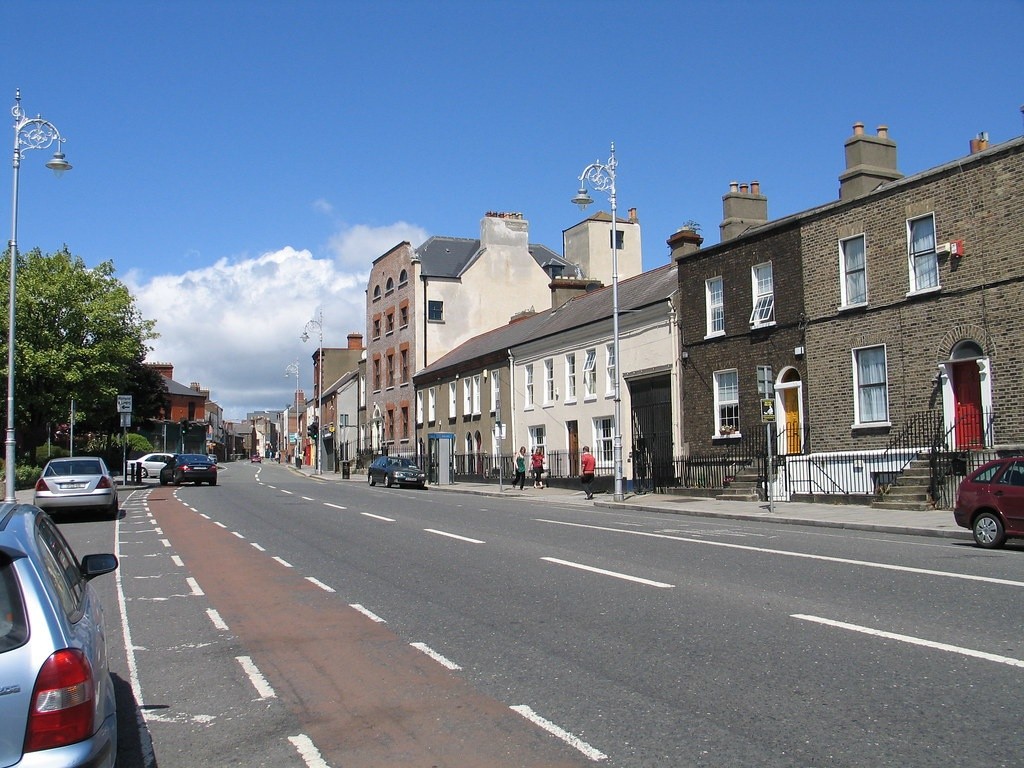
[117,395,132,412]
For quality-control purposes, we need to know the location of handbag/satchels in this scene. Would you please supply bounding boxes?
[541,466,544,473]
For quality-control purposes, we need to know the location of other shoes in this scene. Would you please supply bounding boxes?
[585,492,593,499]
[513,483,515,488]
[541,486,544,489]
[520,487,524,491]
[534,486,537,489]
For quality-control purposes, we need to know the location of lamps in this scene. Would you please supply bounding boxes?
[794,343,805,357]
[483,369,487,378]
[455,373,459,378]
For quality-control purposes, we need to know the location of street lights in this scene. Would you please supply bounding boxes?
[300,310,323,475]
[4,88,73,505]
[284,356,300,458]
[570,141,624,502]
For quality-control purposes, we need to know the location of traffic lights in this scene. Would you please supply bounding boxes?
[180,419,188,435]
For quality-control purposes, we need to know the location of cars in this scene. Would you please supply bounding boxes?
[160,454,217,486]
[0,502,119,768]
[368,456,427,488]
[33,457,118,515]
[126,453,175,478]
[954,457,1024,548]
[251,455,261,463]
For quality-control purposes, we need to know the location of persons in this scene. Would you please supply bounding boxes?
[581,446,595,499]
[277,452,281,464]
[512,446,526,490]
[285,451,289,463]
[530,448,545,489]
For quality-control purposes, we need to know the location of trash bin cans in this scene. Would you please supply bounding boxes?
[342,461,350,479]
